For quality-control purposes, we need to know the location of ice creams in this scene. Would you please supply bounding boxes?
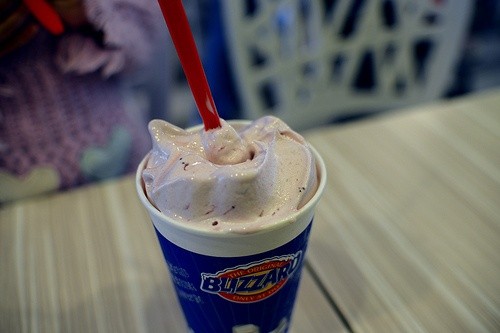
[142,116,318,234]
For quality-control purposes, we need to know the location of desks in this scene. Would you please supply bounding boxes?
[0,84,500,333]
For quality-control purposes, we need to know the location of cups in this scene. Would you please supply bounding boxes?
[134,118,328,333]
